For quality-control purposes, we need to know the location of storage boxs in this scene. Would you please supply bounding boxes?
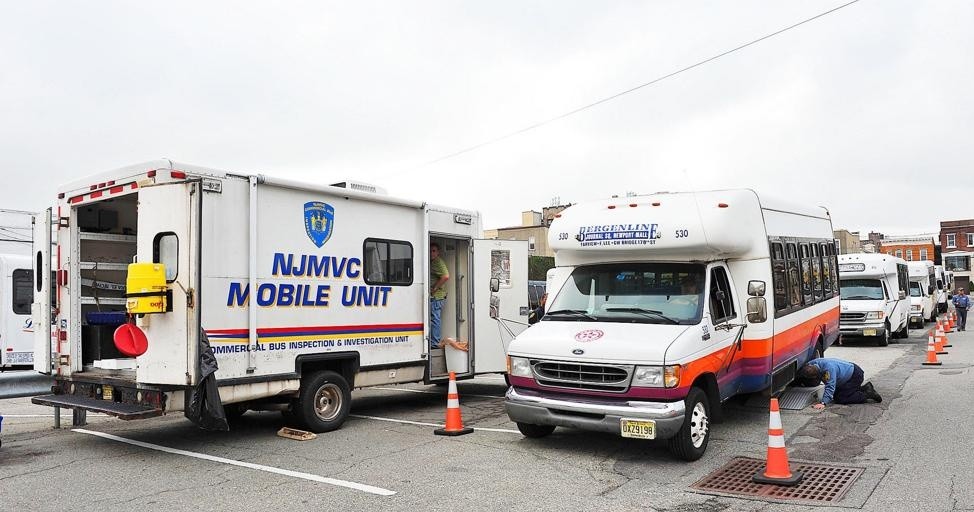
[81,324,129,363]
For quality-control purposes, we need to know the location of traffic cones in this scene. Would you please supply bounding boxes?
[751,399,804,483]
[922,331,942,366]
[435,372,473,435]
[934,307,958,355]
[949,329,950,330]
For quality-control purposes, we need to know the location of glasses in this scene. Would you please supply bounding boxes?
[430,249,436,253]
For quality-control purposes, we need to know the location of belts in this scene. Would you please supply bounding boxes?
[958,307,966,308]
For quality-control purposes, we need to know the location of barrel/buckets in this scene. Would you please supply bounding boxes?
[124,261,168,315]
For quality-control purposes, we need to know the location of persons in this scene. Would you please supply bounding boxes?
[952,286,970,332]
[794,357,884,410]
[668,274,700,306]
[429,243,448,351]
[531,292,546,324]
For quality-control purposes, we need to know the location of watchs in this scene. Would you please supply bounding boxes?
[820,401,827,406]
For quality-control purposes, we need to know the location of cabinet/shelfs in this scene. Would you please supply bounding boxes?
[79,232,137,305]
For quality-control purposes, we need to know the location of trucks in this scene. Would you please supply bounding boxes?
[935,265,950,315]
[908,261,935,327]
[30,161,530,432]
[506,190,842,462]
[946,271,956,299]
[837,249,909,346]
[0,253,59,371]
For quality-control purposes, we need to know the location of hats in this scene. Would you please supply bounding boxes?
[680,275,696,285]
[958,287,964,291]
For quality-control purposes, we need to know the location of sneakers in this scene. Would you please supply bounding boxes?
[962,327,965,331]
[957,328,960,331]
[863,382,882,402]
